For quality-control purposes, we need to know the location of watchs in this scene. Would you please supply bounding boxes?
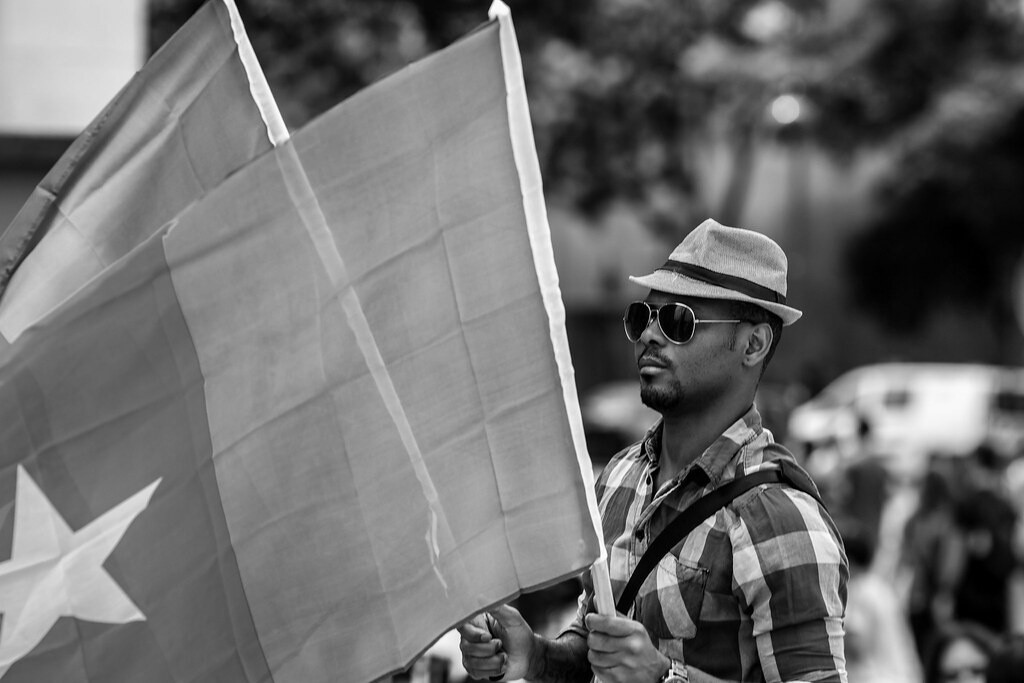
[660,658,689,683]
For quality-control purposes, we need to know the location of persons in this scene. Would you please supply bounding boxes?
[453,220,850,683]
[847,415,1022,682]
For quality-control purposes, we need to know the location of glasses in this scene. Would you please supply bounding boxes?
[622,301,762,345]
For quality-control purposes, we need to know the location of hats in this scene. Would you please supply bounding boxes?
[628,218,803,328]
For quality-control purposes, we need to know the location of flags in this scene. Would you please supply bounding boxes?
[0,0,601,683]
